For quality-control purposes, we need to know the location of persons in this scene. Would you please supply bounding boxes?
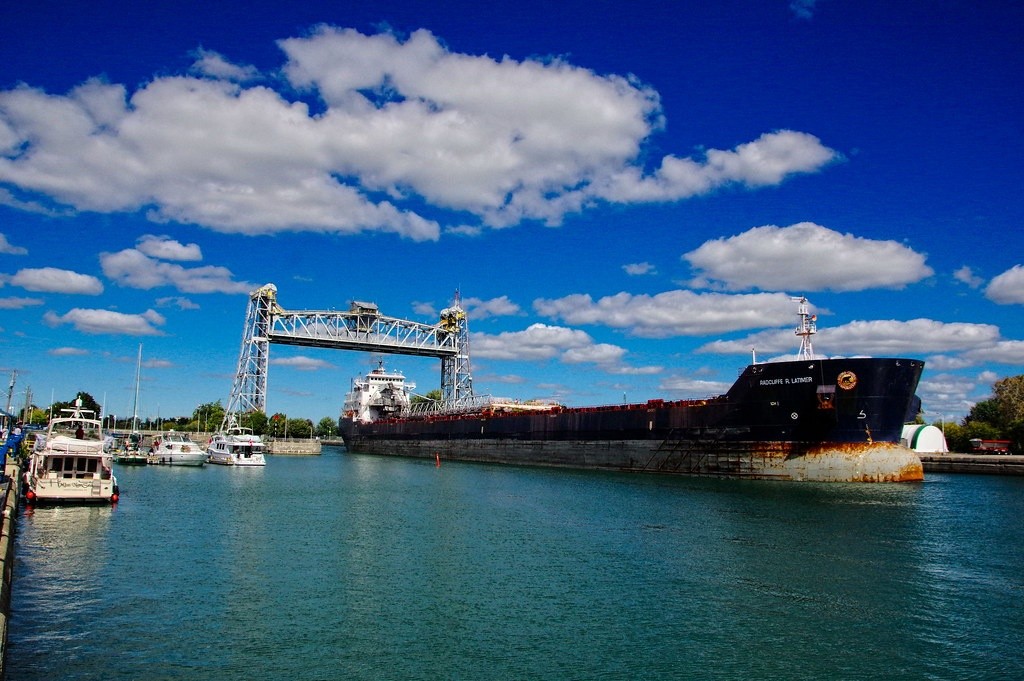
[75,425,85,440]
[1,426,9,441]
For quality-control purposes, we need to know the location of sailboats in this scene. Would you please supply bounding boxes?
[110,340,149,464]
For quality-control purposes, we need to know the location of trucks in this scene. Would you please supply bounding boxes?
[969,438,1014,456]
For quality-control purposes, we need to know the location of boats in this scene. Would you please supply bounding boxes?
[207,420,267,466]
[149,432,211,466]
[22,395,119,501]
[339,358,926,482]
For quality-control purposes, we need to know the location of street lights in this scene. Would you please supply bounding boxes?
[327,426,331,431]
[309,426,312,439]
[936,412,945,451]
